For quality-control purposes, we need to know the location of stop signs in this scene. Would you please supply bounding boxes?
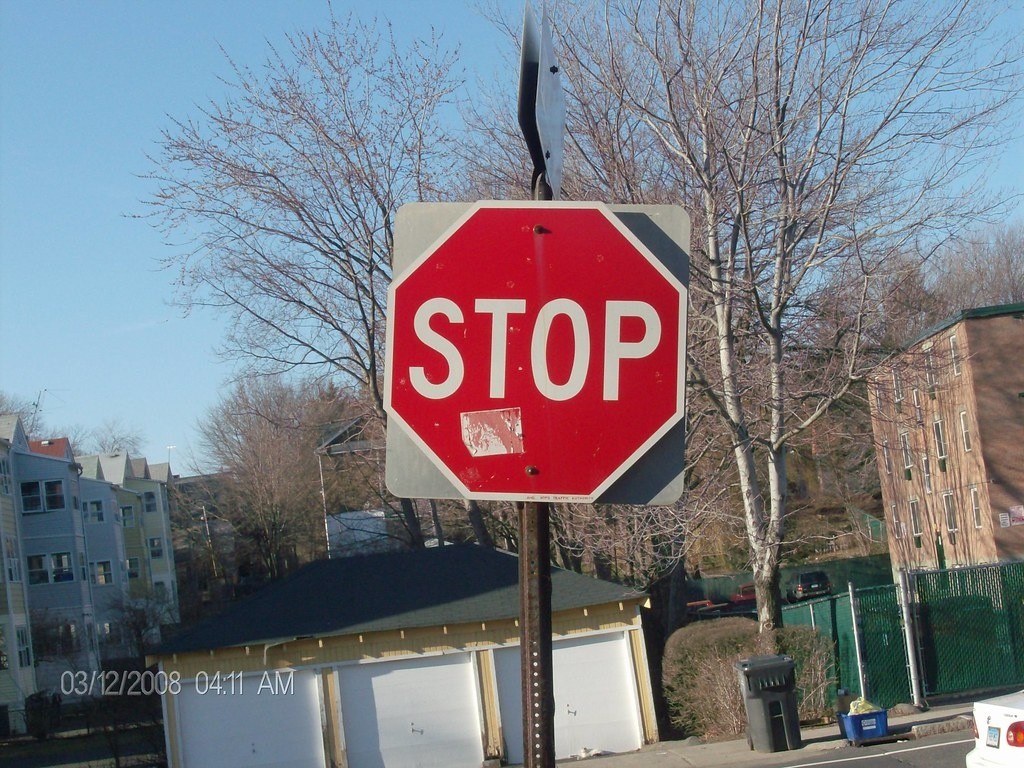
[381,200,687,501]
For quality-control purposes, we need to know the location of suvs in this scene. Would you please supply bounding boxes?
[786,570,832,603]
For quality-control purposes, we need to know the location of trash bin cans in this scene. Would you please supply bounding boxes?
[735,653,802,752]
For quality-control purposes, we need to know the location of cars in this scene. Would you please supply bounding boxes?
[730,583,758,609]
[686,600,730,619]
[966,689,1024,768]
